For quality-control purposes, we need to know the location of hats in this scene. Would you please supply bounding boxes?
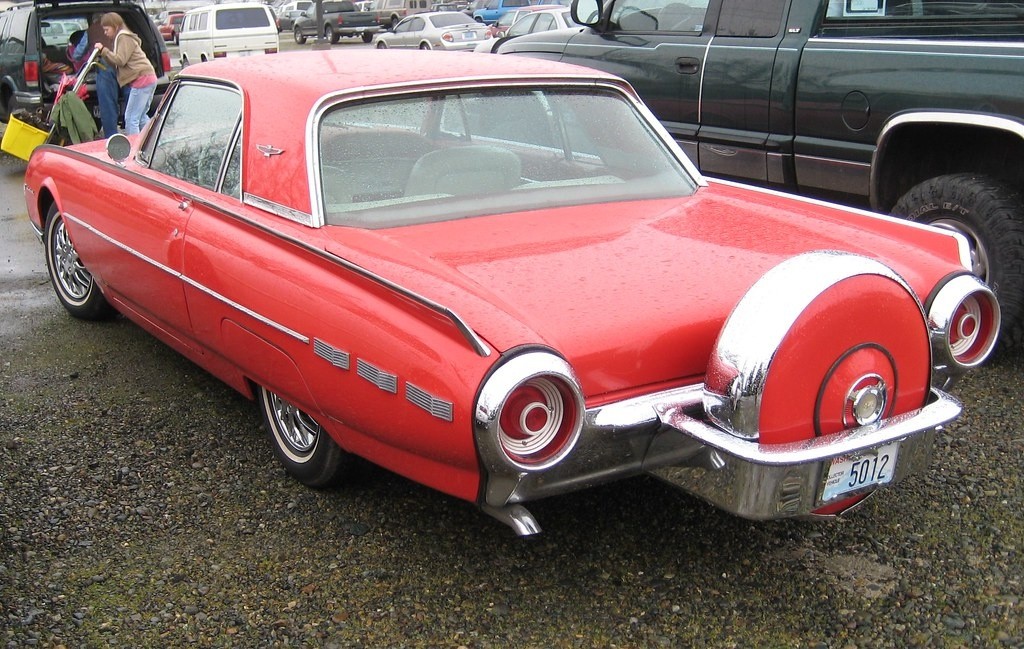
[91,12,104,19]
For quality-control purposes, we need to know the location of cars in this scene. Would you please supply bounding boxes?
[355,0,570,33]
[374,10,487,50]
[263,0,314,34]
[154,10,184,45]
[474,9,583,60]
[23,50,1003,540]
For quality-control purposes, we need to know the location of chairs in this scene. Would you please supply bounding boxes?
[403,146,521,198]
[230,164,347,203]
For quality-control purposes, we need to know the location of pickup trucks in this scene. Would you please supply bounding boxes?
[490,0,1024,314]
[294,0,381,45]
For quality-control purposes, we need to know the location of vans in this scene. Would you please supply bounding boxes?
[178,2,280,70]
[40,21,83,46]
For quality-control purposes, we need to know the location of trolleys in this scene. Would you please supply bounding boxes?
[1,46,106,162]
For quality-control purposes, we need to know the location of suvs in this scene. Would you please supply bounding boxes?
[0,0,174,123]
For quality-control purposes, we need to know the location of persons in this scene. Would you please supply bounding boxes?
[73,13,131,139]
[95,12,157,136]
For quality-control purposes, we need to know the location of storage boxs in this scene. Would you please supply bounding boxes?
[0,114,50,162]
